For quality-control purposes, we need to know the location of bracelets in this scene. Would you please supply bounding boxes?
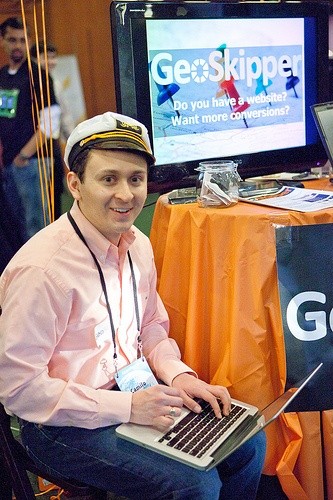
[19,153,29,161]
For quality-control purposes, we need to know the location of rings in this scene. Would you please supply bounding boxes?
[171,408,176,415]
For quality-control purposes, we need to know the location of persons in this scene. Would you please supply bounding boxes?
[0,16,61,277]
[30,41,73,220]
[0,113,268,500]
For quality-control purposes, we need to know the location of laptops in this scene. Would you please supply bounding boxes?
[115,362,324,472]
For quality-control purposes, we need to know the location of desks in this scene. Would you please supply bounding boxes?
[149,175,333,500]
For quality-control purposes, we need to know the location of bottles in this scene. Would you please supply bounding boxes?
[196,160,240,206]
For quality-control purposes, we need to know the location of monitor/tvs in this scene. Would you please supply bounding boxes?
[110,0,319,193]
[310,101,333,167]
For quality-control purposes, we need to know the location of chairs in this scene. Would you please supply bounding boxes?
[0,402,98,500]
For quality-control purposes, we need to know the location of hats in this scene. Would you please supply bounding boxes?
[64,112,156,172]
